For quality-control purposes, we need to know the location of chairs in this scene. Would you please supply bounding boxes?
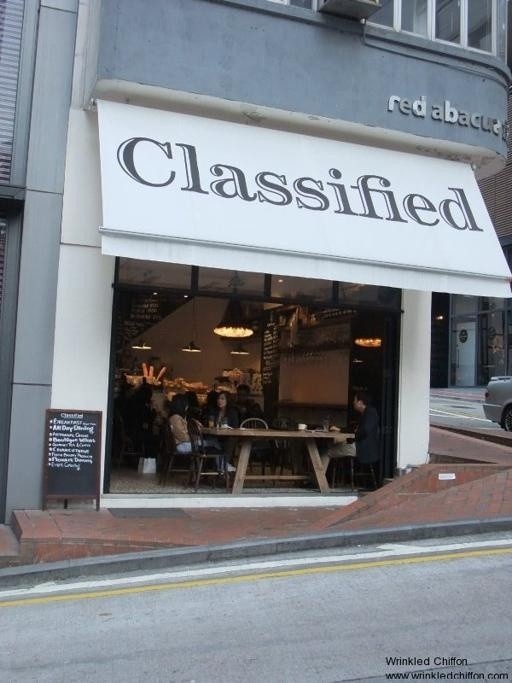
[112,405,380,493]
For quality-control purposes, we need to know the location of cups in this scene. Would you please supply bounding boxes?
[298,423,307,430]
[208,416,228,430]
[323,417,330,433]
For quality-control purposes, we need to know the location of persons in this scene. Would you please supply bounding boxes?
[295,390,383,490]
[120,379,264,473]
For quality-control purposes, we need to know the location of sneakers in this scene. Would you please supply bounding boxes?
[223,462,236,472]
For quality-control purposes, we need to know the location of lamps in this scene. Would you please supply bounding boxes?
[231,338,249,355]
[181,294,202,353]
[355,309,382,347]
[213,269,254,338]
[131,292,153,350]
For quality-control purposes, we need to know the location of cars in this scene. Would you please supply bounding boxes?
[483,376,512,431]
